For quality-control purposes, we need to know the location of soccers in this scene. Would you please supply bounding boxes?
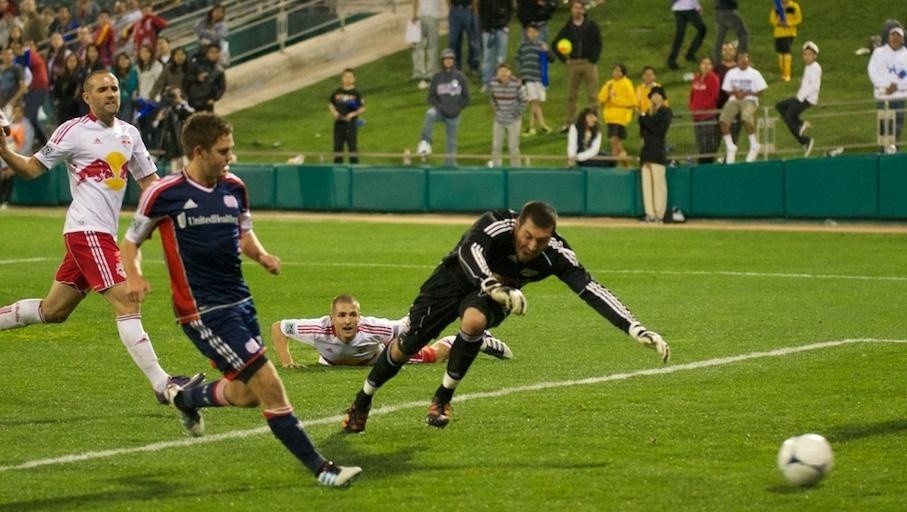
[557,39,572,55]
[776,432,833,485]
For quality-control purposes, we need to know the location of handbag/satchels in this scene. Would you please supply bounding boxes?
[405,17,423,46]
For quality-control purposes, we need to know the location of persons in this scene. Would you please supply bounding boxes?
[340,200,672,433]
[867,20,907,154]
[1,1,228,204]
[329,68,366,164]
[670,0,823,161]
[406,0,604,168]
[566,62,673,224]
[120,112,363,488]
[271,294,513,369]
[0,69,207,407]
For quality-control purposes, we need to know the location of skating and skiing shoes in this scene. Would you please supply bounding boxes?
[541,126,551,134]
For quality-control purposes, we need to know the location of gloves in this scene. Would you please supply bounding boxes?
[488,282,532,315]
[626,320,673,363]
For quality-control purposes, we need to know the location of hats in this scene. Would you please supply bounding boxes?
[648,87,668,101]
[440,48,459,60]
[802,41,821,55]
[888,26,905,37]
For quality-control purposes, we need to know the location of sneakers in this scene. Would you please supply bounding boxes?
[521,129,537,138]
[723,145,737,166]
[801,135,815,157]
[314,457,363,489]
[743,144,760,164]
[416,140,432,157]
[475,327,516,360]
[164,381,207,437]
[344,399,370,433]
[154,372,207,404]
[425,394,452,427]
[796,117,809,136]
